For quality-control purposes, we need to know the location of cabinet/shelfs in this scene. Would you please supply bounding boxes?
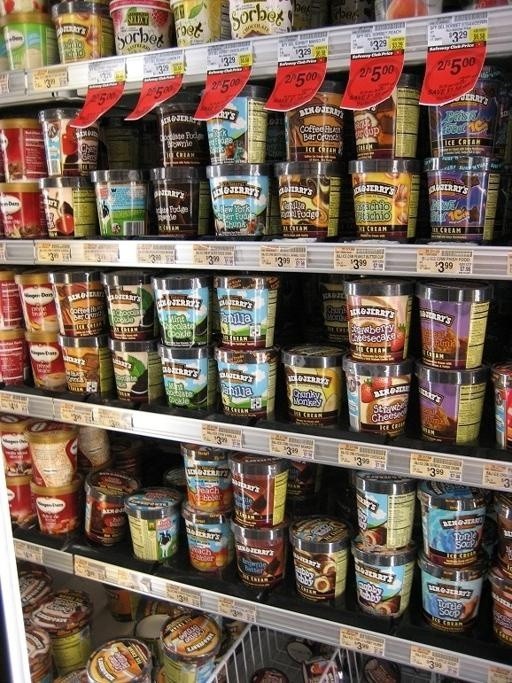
[1,6,512,682]
